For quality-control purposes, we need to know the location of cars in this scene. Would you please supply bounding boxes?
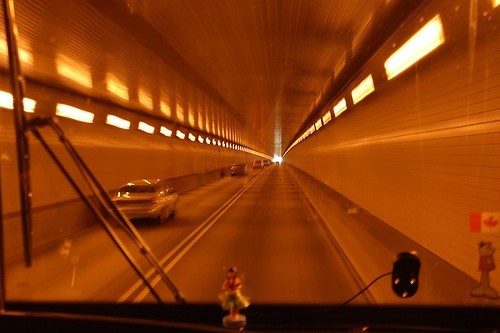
[231,163,247,176]
[112,180,178,223]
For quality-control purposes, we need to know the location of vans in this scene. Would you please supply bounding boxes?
[253,159,270,170]
